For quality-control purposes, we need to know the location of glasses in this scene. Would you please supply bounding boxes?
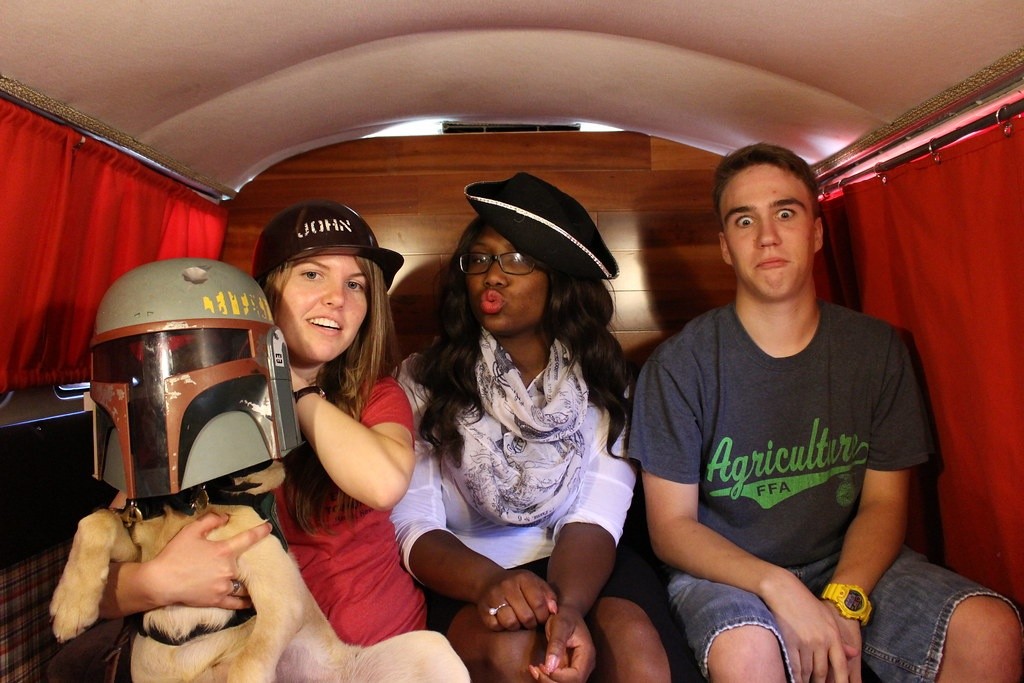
[459,250,549,275]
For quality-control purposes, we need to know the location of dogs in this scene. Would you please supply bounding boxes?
[49,461,471,683]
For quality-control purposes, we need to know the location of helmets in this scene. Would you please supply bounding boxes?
[253,199,404,296]
[90,258,305,499]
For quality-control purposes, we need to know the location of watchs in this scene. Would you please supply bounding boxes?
[294,385,327,404]
[821,583,872,625]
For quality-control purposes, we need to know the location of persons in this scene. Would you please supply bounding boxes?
[626,143,1024,683]
[391,172,676,682]
[99,197,426,683]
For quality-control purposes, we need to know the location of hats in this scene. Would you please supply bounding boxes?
[464,172,620,281]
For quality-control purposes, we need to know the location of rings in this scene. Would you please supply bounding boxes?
[231,580,239,596]
[489,604,506,616]
